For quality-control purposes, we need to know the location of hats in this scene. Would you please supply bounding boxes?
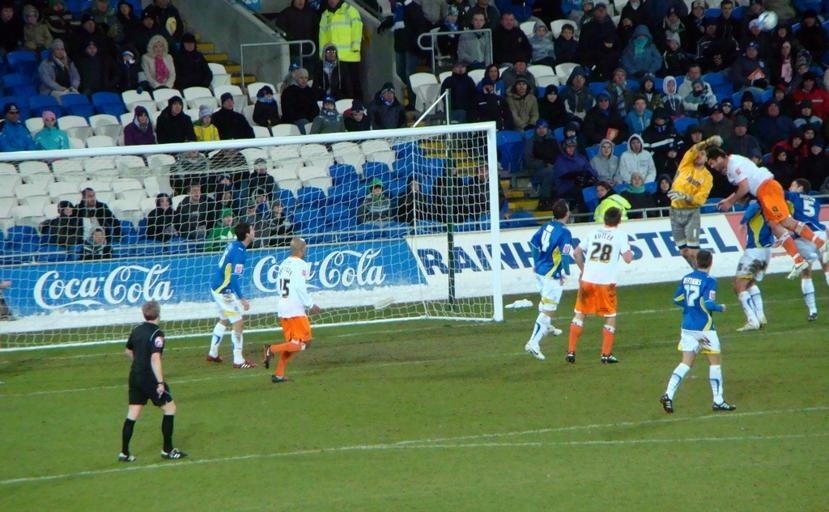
[42,111,55,121]
[198,105,212,122]
[741,91,755,105]
[562,138,578,152]
[321,41,342,89]
[140,9,154,21]
[748,19,762,31]
[691,0,705,9]
[535,120,549,128]
[51,39,63,50]
[732,115,748,128]
[3,103,19,114]
[81,12,95,23]
[666,32,680,46]
[799,99,812,109]
[49,0,64,10]
[379,82,395,95]
[323,97,335,104]
[84,41,98,49]
[662,75,677,112]
[220,92,233,103]
[632,91,648,105]
[631,172,644,185]
[351,100,364,113]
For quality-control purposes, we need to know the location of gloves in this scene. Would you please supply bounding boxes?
[666,190,690,201]
[705,135,723,147]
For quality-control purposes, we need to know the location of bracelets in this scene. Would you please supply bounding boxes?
[157,382,164,384]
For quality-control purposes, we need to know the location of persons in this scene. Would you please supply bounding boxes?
[659,248,736,413]
[207,223,258,369]
[565,207,632,363]
[667,135,723,275]
[524,199,573,361]
[118,301,186,462]
[733,195,775,331]
[706,148,829,280]
[1,1,829,261]
[782,179,829,321]
[262,237,319,383]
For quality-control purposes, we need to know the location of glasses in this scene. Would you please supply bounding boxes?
[8,111,19,115]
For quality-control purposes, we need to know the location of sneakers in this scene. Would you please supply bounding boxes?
[117,452,136,463]
[160,447,186,460]
[759,318,768,329]
[807,309,818,321]
[232,360,258,368]
[787,258,808,281]
[524,342,545,360]
[565,352,575,364]
[712,401,736,410]
[547,325,562,336]
[207,355,223,362]
[271,375,288,382]
[263,344,274,368]
[659,394,674,414]
[600,352,619,363]
[816,239,828,265]
[734,321,760,332]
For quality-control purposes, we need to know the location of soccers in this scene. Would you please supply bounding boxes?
[759,10,778,31]
